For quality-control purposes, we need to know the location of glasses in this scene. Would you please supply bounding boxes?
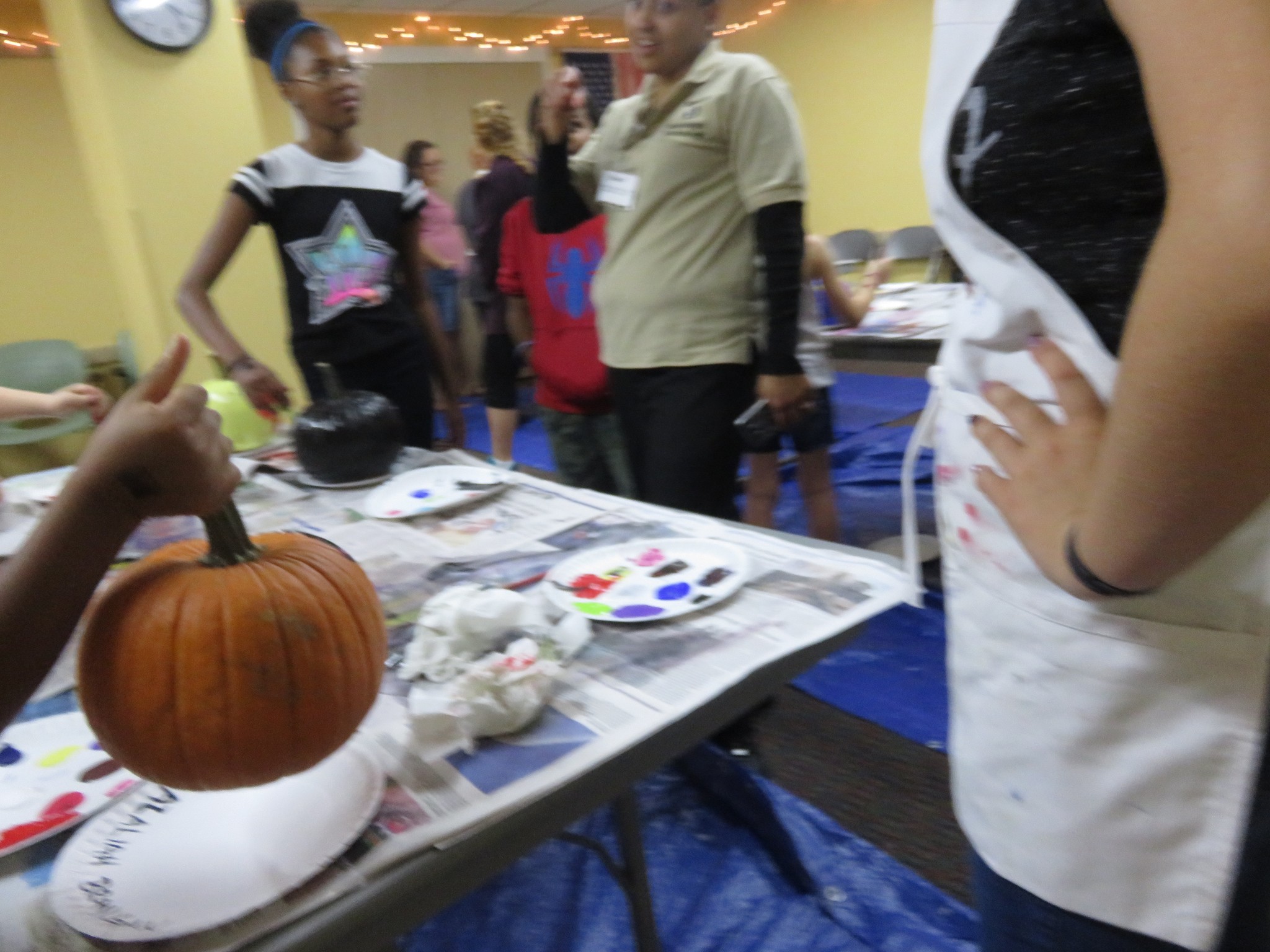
[285,64,373,86]
[421,160,444,166]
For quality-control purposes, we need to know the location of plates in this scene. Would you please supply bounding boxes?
[543,538,754,623]
[48,739,386,945]
[0,708,142,858]
[364,465,514,518]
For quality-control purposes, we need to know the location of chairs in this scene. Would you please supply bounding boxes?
[0,338,108,456]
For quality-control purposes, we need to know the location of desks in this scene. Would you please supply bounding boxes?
[814,275,965,383]
[3,429,937,952]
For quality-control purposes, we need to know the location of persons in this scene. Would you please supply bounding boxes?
[0,380,113,426]
[743,234,891,539]
[459,100,533,471]
[173,0,465,452]
[532,0,812,525]
[498,83,638,503]
[402,139,484,411]
[921,0,1270,950]
[0,335,241,739]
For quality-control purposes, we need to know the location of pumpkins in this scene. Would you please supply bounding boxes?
[77,492,388,792]
[293,361,408,481]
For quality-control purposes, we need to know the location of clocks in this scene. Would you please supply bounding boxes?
[110,0,218,52]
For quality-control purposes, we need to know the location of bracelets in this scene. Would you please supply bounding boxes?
[1065,525,1157,598]
[223,353,253,381]
